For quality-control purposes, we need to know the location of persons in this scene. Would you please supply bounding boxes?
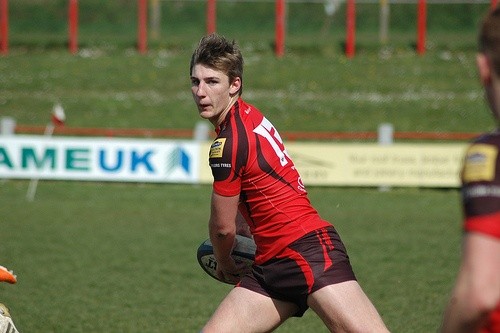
[190,33,389,333]
[438,0,500,333]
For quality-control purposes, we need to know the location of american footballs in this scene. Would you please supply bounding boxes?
[196,234,257,287]
